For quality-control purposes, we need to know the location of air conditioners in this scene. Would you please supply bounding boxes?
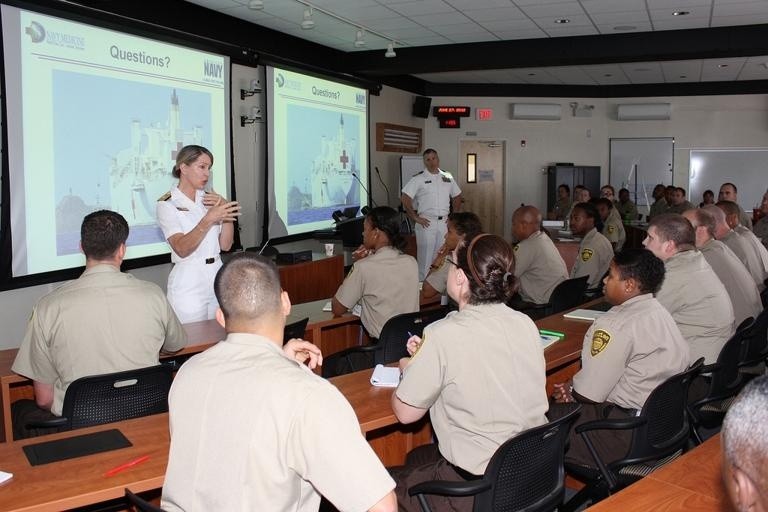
[617,102,671,120]
[512,103,562,120]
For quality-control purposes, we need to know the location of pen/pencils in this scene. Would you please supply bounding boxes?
[408,331,412,337]
[539,329,564,337]
[107,455,152,478]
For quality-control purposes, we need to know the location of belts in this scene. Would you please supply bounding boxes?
[205,258,216,264]
[446,460,483,482]
[603,401,637,418]
[437,215,444,221]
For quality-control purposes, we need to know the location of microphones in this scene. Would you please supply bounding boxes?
[374,166,389,206]
[351,172,378,208]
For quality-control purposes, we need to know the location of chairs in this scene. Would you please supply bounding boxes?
[340,309,448,372]
[556,358,705,512]
[527,275,590,321]
[689,317,758,446]
[408,401,582,512]
[25,363,174,436]
[283,316,309,346]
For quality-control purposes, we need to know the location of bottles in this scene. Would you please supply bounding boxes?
[564,215,570,231]
[624,210,632,225]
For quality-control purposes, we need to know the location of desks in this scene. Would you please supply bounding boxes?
[0,296,610,511]
[0,280,443,444]
[583,433,735,511]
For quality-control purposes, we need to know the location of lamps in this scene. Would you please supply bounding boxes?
[354,28,366,48]
[301,6,314,29]
[385,41,396,57]
[248,0,265,10]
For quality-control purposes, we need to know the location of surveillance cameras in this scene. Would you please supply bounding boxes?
[249,78,260,91]
[249,106,260,119]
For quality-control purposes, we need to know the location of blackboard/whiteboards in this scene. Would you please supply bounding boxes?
[688,150,768,213]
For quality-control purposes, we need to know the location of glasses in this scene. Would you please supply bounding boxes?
[600,192,614,197]
[442,254,472,280]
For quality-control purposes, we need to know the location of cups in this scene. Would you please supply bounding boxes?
[324,244,334,256]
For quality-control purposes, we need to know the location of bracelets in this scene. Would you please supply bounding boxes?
[430,264,440,269]
[569,384,577,403]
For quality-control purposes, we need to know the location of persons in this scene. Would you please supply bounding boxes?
[649,183,669,221]
[321,205,423,377]
[566,185,585,219]
[401,149,463,281]
[718,182,753,232]
[668,187,696,214]
[601,184,625,223]
[566,187,593,231]
[508,205,570,321]
[641,212,737,385]
[664,185,677,213]
[422,211,486,317]
[715,200,768,275]
[681,208,768,328]
[543,246,690,464]
[618,187,639,220]
[696,189,718,210]
[719,373,768,512]
[378,232,551,510]
[752,188,768,251]
[161,250,402,511]
[596,198,626,256]
[699,204,768,311]
[568,201,614,303]
[10,210,187,440]
[154,145,243,325]
[551,184,571,221]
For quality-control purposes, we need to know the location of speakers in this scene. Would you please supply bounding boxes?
[411,96,432,118]
